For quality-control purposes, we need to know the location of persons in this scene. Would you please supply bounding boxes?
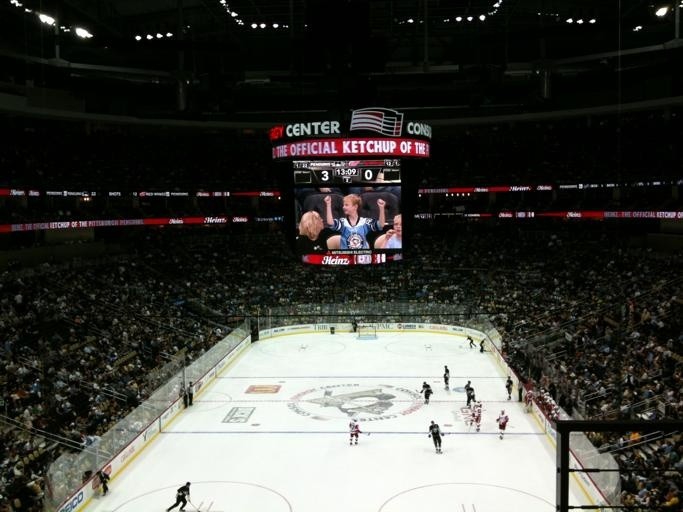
[444,365,450,389]
[491,334,683,512]
[349,418,361,445]
[2,213,683,333]
[466,335,486,352]
[465,380,482,432]
[429,420,444,454]
[1,333,231,511]
[420,381,433,404]
[296,195,403,249]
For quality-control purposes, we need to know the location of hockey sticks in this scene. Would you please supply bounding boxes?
[359,431,372,437]
